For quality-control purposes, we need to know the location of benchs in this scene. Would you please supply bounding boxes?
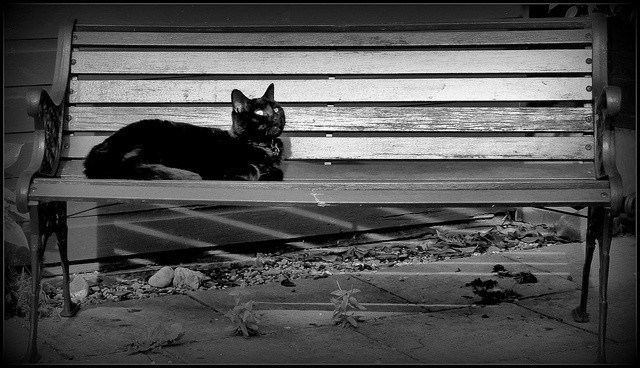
[16,23,625,363]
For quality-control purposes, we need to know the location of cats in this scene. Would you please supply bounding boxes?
[82,82,286,181]
